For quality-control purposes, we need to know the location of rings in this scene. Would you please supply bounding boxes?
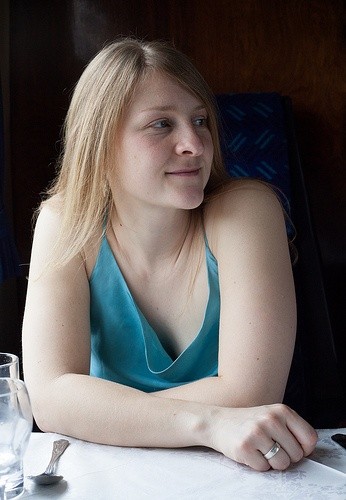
[262,441,281,460]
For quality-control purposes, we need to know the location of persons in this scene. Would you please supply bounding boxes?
[22,36,318,470]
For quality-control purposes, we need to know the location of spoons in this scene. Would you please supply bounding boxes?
[26,439,70,484]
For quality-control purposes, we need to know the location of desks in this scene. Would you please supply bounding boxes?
[16,428,346,500]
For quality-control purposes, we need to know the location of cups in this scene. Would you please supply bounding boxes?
[0,353,33,500]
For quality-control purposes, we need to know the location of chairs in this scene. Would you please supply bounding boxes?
[210,93,346,429]
